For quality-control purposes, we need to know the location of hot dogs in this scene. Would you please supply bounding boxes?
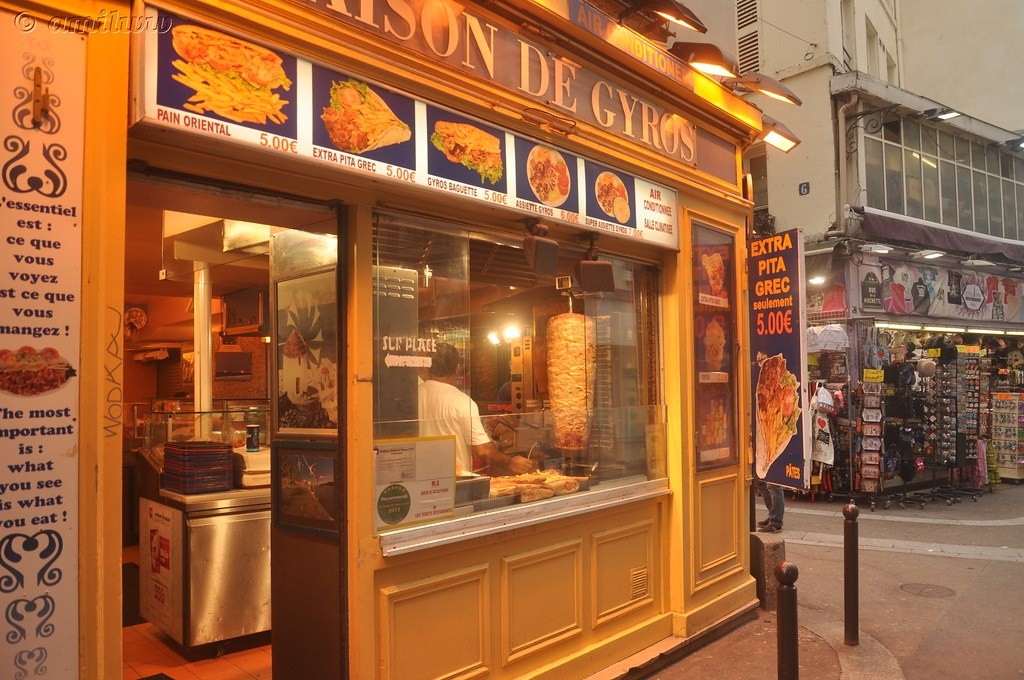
[172,25,292,91]
[431,121,503,184]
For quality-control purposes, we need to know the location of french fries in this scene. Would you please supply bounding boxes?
[172,59,293,125]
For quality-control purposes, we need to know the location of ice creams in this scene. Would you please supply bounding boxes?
[703,318,726,371]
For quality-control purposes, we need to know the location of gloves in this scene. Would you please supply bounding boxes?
[885,392,929,483]
[885,362,916,387]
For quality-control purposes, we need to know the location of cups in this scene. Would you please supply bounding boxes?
[245,425,260,451]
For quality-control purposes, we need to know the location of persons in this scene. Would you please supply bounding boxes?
[418,342,533,477]
[757,480,785,533]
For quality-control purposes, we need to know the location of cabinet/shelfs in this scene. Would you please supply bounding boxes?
[139,488,272,658]
[860,354,1024,512]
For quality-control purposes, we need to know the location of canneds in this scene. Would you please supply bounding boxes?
[246,425,260,452]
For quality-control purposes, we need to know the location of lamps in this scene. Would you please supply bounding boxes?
[913,107,964,123]
[960,259,996,268]
[859,244,894,254]
[618,0,708,39]
[871,320,1024,335]
[908,250,946,261]
[991,136,1024,153]
[759,114,802,154]
[708,71,803,107]
[668,41,742,78]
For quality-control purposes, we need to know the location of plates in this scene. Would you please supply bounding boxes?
[527,145,571,207]
[595,171,629,217]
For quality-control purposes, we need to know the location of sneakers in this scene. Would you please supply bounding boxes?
[757,517,783,526]
[757,523,783,533]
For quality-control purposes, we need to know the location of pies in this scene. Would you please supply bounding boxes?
[756,354,802,479]
[320,77,411,154]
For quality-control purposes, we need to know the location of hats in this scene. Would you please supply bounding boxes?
[918,358,936,379]
